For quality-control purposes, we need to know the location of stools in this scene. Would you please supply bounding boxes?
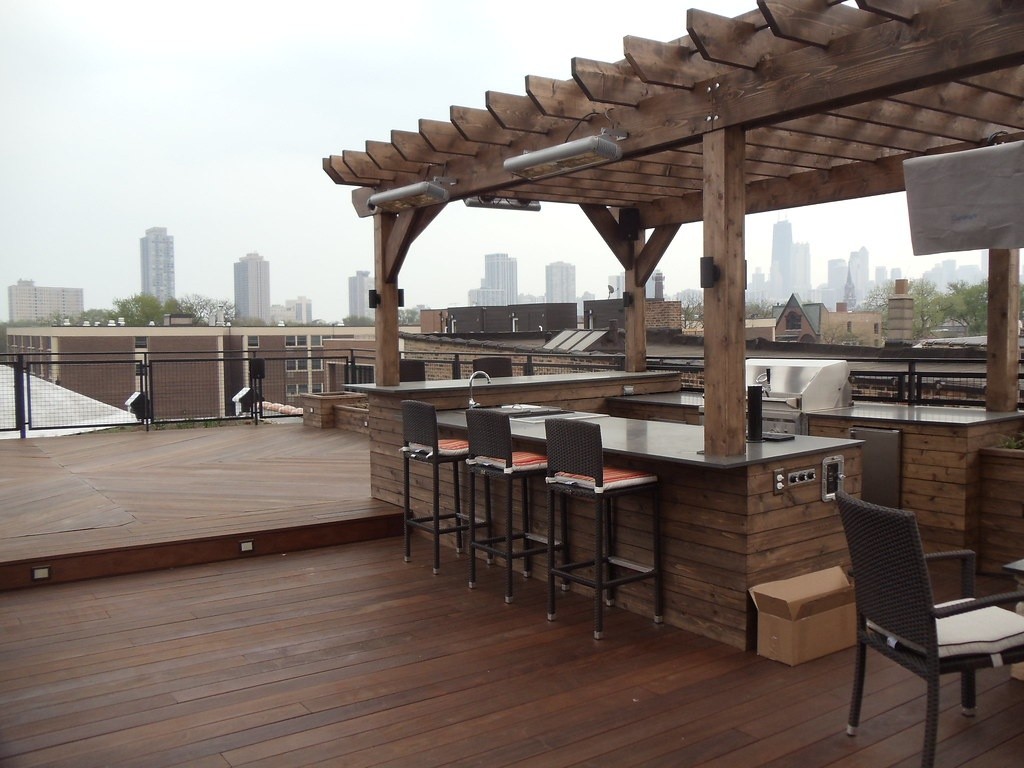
[464,408,570,603]
[399,358,426,382]
[401,399,494,575]
[472,357,512,379]
[544,418,665,639]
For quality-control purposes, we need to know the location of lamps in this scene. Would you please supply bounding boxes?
[700,257,721,289]
[622,292,633,308]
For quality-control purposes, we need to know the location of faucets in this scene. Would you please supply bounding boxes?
[468,370,492,409]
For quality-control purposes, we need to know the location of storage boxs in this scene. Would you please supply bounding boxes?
[749,566,857,667]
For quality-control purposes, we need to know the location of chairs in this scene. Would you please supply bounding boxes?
[836,490,1024,768]
